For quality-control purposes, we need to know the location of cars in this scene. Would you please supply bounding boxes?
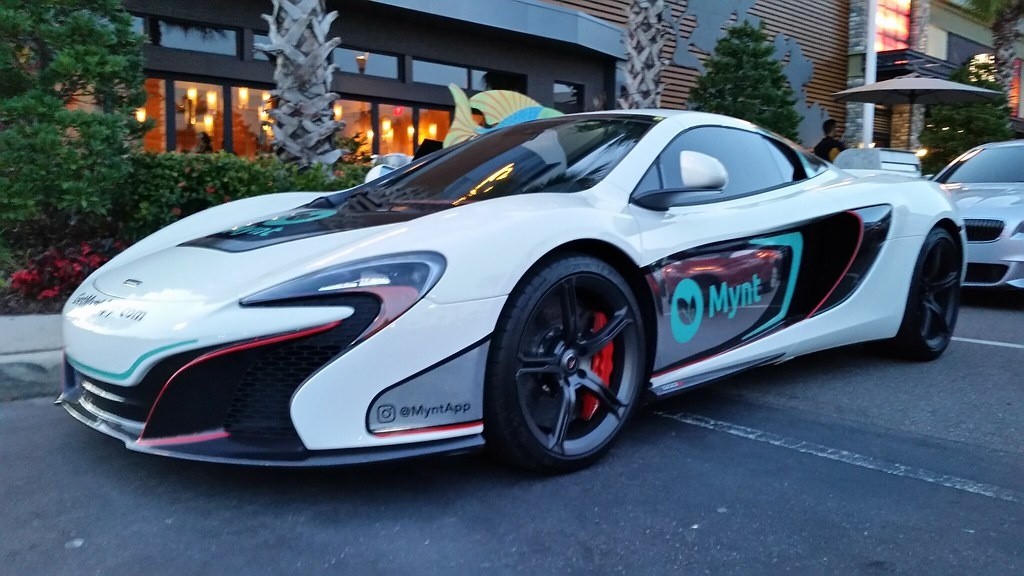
[926,139,1024,292]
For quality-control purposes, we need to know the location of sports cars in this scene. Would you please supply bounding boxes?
[58,108,965,466]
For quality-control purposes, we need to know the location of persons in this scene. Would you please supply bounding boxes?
[195,132,213,154]
[814,119,845,164]
[646,274,664,318]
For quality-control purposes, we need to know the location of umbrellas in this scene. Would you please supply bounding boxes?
[832,72,1005,149]
